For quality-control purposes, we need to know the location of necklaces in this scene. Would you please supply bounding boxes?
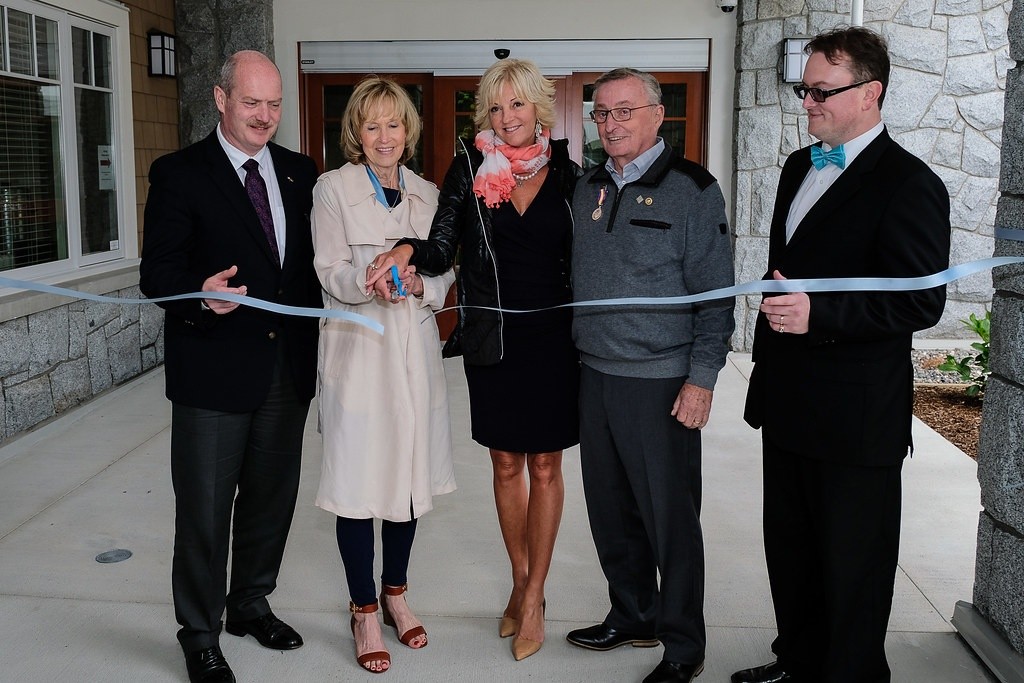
[512,170,538,186]
[378,189,400,213]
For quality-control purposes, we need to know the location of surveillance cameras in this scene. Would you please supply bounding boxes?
[715,0,737,13]
[493,48,511,60]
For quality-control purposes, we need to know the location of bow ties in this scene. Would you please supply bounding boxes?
[810,144,846,170]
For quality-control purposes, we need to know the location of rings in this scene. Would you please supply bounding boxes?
[369,263,378,270]
[694,420,702,424]
[780,315,784,325]
[779,325,784,333]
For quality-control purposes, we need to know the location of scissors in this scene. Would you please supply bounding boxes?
[388,265,409,303]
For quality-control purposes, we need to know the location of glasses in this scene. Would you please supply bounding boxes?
[792,80,872,103]
[588,103,655,124]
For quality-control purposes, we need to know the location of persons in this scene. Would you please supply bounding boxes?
[310,78,457,673]
[730,26,950,683]
[139,50,325,682]
[363,56,585,659]
[566,72,735,683]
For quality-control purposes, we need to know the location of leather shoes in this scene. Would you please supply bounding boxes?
[565,622,660,650]
[184,646,236,683]
[731,661,797,683]
[642,659,704,683]
[225,613,304,650]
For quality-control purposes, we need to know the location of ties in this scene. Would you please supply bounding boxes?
[242,159,281,270]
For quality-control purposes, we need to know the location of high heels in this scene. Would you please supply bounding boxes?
[500,610,519,637]
[378,583,428,649]
[511,598,547,660]
[349,598,391,674]
